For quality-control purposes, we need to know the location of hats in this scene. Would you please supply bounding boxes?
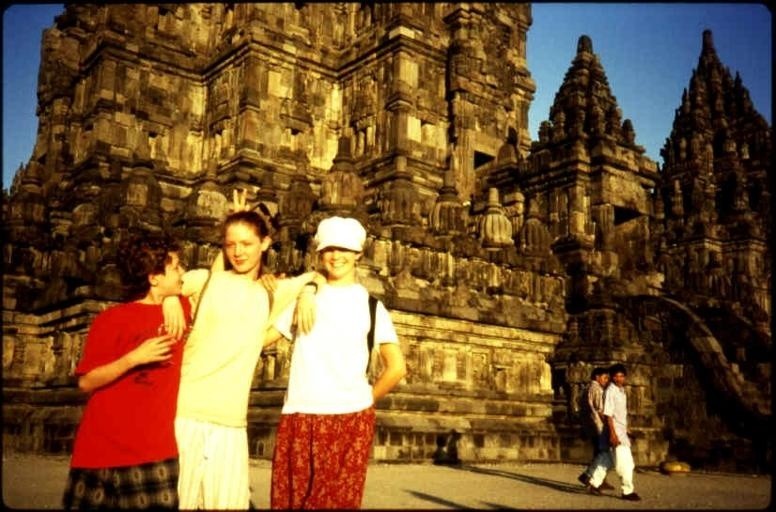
[314,216,366,252]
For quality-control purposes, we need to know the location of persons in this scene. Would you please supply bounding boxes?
[161,211,328,510]
[262,215,407,510]
[577,367,615,491]
[586,364,642,501]
[63,223,197,512]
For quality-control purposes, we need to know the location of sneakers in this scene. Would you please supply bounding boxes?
[621,493,641,501]
[578,472,615,495]
